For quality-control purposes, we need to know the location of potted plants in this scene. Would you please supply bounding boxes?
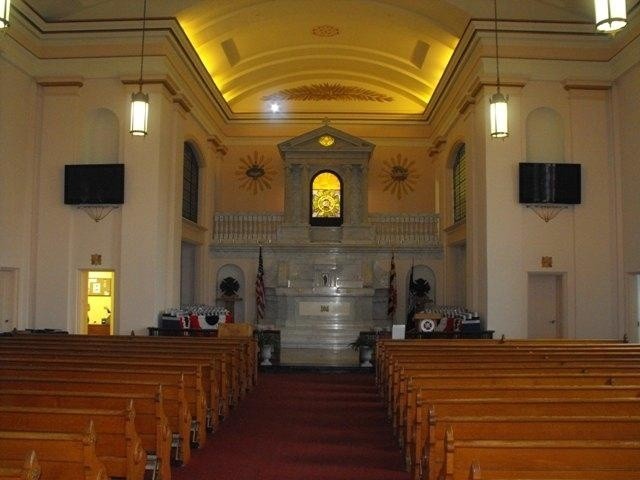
[348,334,375,368]
[257,333,282,366]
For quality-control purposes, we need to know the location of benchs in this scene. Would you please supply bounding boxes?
[1,332,259,480]
[374,336,640,480]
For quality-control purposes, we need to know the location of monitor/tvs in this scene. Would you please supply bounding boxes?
[519,162,581,204]
[64,163,125,204]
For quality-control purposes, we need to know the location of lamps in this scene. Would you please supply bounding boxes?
[128,0,150,136]
[488,1,511,140]
[593,0,629,35]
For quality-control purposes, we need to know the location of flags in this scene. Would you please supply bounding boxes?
[253,245,267,323]
[388,252,398,319]
